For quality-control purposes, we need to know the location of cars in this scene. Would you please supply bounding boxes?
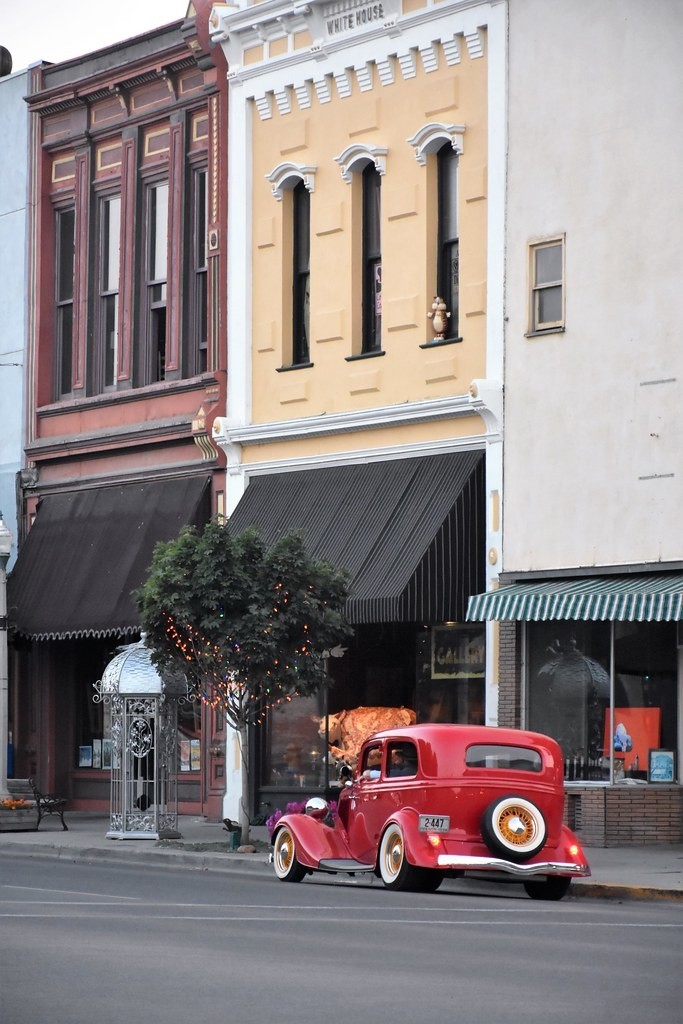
[270,723,593,899]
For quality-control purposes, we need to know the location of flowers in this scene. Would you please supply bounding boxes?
[0,798,36,810]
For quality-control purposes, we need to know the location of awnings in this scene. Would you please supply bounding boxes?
[5,475,212,641]
[465,572,683,622]
[225,449,486,627]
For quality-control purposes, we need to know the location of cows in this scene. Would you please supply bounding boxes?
[310,705,418,773]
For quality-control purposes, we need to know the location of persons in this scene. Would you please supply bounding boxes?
[363,749,410,781]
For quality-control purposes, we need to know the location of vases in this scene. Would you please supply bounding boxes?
[0,809,40,832]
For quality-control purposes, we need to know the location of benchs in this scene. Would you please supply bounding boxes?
[5,778,67,832]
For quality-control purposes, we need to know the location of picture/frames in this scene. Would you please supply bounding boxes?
[648,748,676,783]
